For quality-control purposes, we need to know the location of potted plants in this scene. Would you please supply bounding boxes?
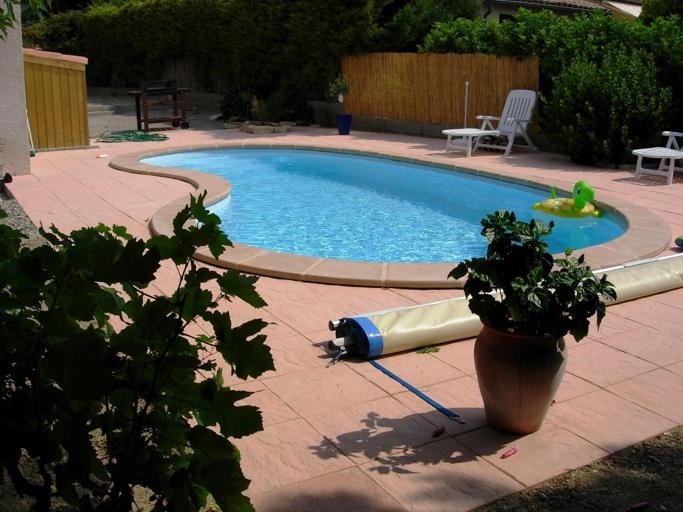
[446,210,616,435]
[328,74,352,135]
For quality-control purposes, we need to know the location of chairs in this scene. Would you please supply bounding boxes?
[441,89,539,158]
[632,130,683,186]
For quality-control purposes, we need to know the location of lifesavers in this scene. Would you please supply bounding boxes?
[532,180,603,218]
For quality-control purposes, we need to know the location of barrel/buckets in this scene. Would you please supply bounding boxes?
[335,111,352,135]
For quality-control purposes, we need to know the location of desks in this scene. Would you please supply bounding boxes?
[127,87,193,133]
